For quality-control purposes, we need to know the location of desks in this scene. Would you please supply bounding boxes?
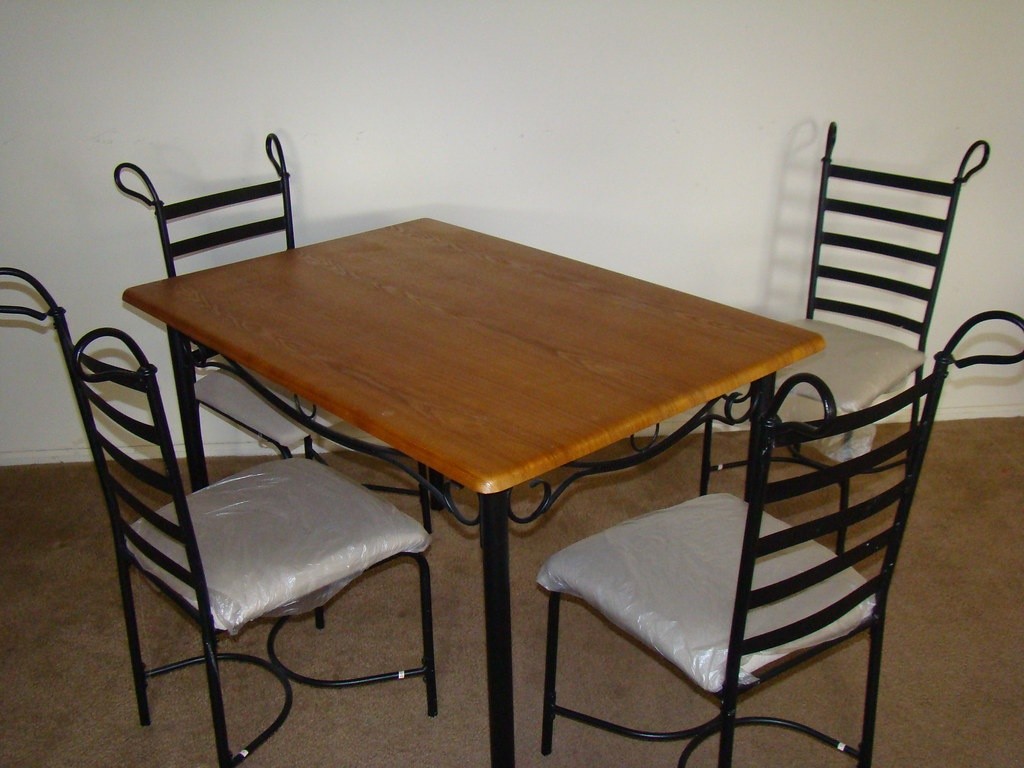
[122,219,827,768]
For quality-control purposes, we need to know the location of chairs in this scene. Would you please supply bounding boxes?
[695,122,989,568]
[113,132,432,537]
[0,268,436,768]
[535,309,1024,768]
